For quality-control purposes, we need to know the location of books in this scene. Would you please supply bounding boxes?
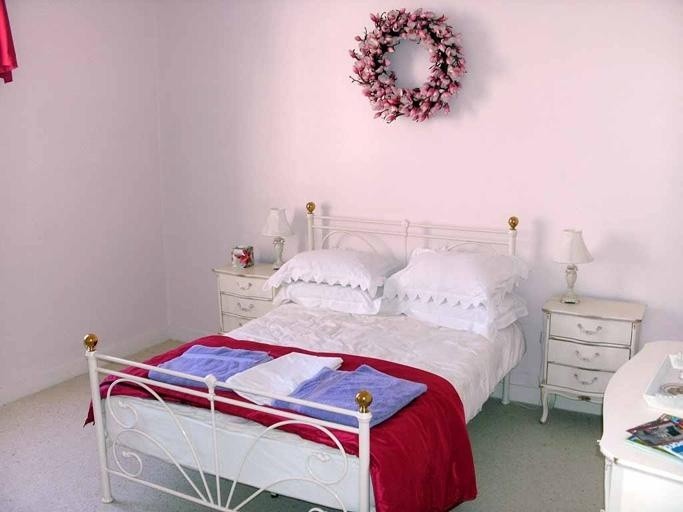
[628,412,683,456]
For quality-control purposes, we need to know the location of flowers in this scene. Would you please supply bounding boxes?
[349,7,465,123]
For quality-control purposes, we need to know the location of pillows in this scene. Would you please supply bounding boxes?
[384,249,532,309]
[381,292,529,342]
[263,248,405,297]
[272,280,385,315]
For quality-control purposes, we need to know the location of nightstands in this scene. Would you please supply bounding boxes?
[213,261,283,332]
[538,296,646,424]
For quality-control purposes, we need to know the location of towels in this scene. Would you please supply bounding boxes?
[148,344,274,394]
[225,352,343,407]
[271,362,428,429]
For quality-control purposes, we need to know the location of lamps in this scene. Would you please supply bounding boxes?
[260,208,295,270]
[552,229,593,304]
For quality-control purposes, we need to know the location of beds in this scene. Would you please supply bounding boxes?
[83,202,519,512]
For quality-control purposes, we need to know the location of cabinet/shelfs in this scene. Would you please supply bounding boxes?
[597,341,683,512]
